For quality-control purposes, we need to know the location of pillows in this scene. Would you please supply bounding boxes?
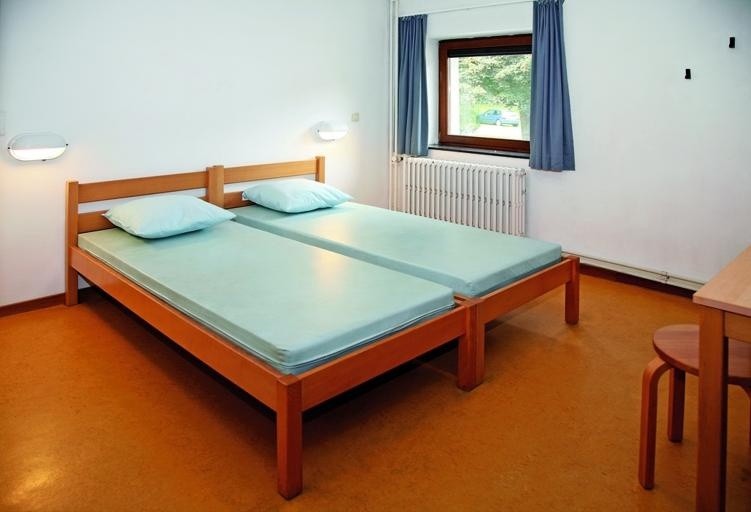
[242,178,353,213]
[101,195,238,238]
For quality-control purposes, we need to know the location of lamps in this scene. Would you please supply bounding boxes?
[316,119,348,141]
[6,133,69,161]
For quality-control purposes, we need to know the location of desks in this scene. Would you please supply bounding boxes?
[692,244,751,511]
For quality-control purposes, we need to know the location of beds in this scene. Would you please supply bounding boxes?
[66,167,467,501]
[216,158,581,389]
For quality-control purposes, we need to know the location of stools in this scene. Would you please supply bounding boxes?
[638,322,751,490]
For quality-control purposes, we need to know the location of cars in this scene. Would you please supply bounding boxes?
[477,108,520,127]
